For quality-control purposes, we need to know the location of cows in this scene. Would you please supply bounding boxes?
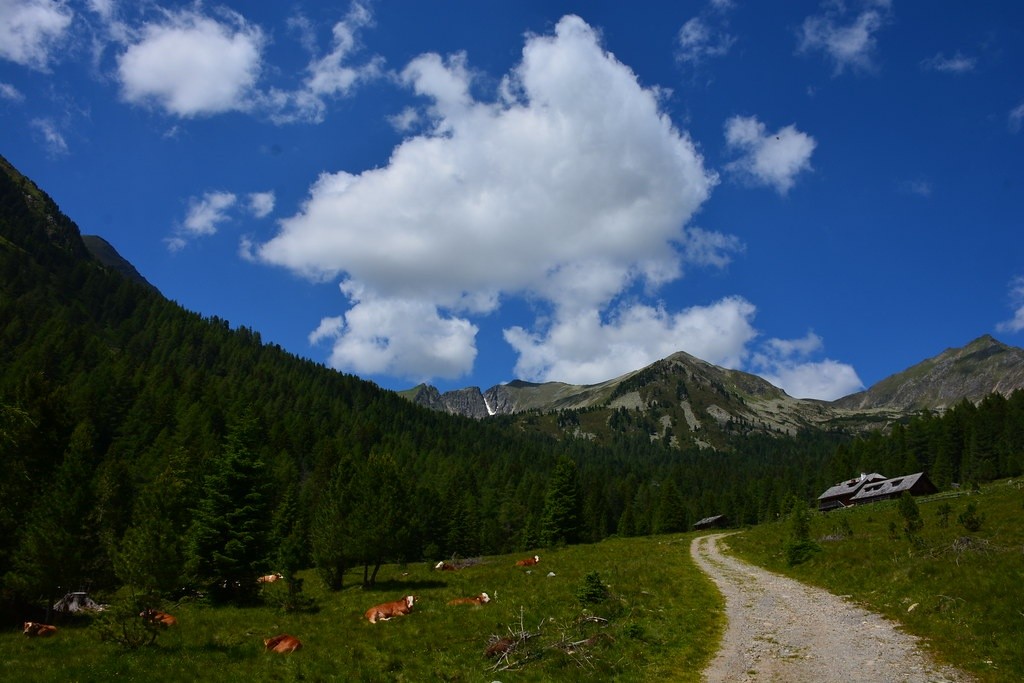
[434,560,463,573]
[139,608,177,628]
[254,571,285,590]
[360,594,420,625]
[446,592,491,609]
[22,621,63,639]
[262,633,304,657]
[515,555,540,567]
